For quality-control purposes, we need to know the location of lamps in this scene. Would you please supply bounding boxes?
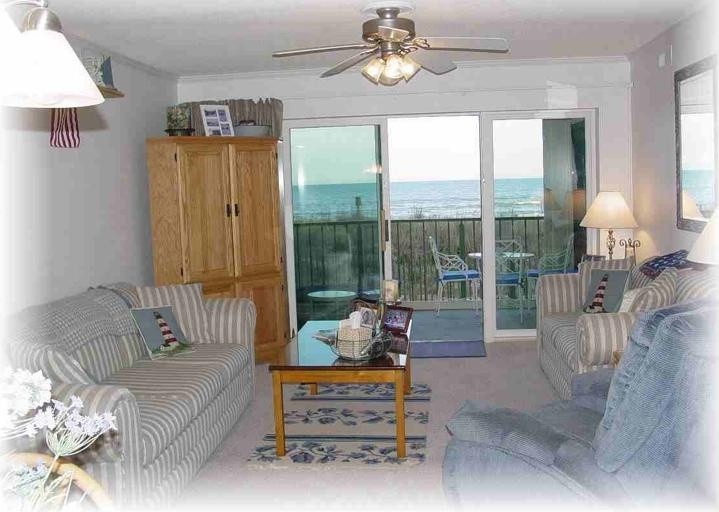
[580,190,640,259]
[1,0,106,110]
[685,202,719,267]
[361,53,423,87]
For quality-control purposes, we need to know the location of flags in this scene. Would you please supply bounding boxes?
[49,107,80,148]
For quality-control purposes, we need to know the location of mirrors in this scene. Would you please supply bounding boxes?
[674,54,717,233]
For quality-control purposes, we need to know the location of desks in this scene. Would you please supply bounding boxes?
[468,252,535,261]
[269,320,413,458]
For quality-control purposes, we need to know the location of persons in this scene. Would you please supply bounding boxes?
[361,310,368,324]
[205,110,232,136]
[387,312,400,325]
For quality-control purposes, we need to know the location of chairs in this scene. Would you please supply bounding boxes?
[527,233,575,316]
[427,235,481,317]
[496,240,523,324]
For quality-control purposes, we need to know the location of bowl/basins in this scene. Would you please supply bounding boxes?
[234,125,271,136]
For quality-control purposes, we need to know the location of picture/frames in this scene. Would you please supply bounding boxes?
[354,300,382,330]
[380,304,413,331]
[199,104,235,136]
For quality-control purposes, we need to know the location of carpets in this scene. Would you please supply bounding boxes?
[244,382,432,469]
[408,340,488,359]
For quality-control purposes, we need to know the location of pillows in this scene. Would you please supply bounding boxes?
[129,305,195,360]
[628,266,678,313]
[577,256,636,312]
[584,269,630,313]
[135,283,217,344]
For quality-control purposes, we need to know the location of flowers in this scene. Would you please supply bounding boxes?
[6,367,117,511]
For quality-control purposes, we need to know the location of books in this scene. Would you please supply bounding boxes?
[312,328,338,345]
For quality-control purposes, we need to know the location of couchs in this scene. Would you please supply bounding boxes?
[5,281,257,512]
[441,299,719,510]
[535,248,719,399]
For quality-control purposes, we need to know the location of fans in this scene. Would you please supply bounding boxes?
[272,0,509,87]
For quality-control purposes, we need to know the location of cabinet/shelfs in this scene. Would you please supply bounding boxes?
[143,137,290,365]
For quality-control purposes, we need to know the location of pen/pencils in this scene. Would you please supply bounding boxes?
[361,331,383,355]
